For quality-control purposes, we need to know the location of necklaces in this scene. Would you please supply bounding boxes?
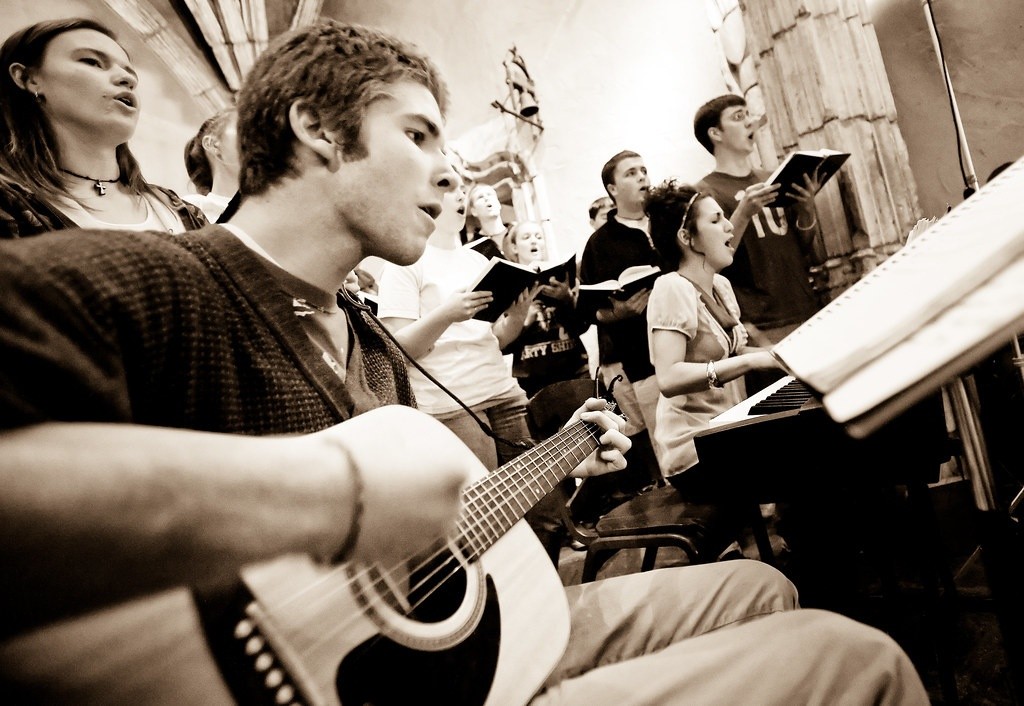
[617,213,646,220]
[56,165,122,196]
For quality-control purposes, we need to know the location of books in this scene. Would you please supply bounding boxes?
[763,148,852,212]
[770,151,1024,432]
[464,236,660,324]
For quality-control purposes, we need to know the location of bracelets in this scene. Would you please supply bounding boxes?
[796,217,817,230]
[706,358,724,390]
[611,309,622,319]
[303,437,365,569]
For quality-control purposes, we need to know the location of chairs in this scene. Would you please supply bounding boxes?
[522,378,775,585]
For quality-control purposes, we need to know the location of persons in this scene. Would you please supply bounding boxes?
[378,164,577,573]
[0,17,211,242]
[694,97,828,396]
[644,184,788,510]
[0,18,931,706]
[178,106,513,314]
[493,220,591,400]
[573,148,708,495]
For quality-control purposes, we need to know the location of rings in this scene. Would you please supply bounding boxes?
[474,307,477,313]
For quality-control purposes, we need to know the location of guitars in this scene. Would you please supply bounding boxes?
[1,374,627,706]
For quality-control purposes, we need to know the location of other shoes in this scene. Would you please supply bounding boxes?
[562,532,598,551]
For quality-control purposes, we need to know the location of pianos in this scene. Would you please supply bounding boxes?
[695,373,942,503]
